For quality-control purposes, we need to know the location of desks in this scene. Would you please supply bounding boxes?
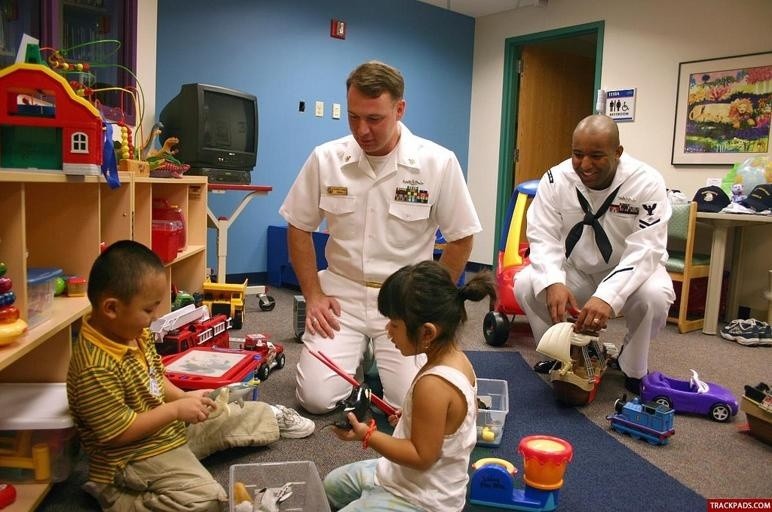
[697,211,772,336]
[208,182,273,285]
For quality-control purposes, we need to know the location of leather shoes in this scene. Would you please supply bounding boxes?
[616,346,646,395]
[535,360,562,372]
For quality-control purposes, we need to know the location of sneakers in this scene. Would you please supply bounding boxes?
[721,320,760,346]
[279,403,315,439]
[758,319,772,346]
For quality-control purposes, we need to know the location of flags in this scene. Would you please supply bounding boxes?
[469,435,574,512]
[239,331,286,379]
[536,319,609,409]
[332,382,372,430]
[160,347,261,403]
[192,276,249,330]
[0,31,193,189]
[148,303,229,354]
[0,261,28,346]
[200,376,261,409]
[637,367,738,422]
[606,393,676,448]
[207,387,231,421]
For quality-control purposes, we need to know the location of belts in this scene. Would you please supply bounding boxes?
[327,267,383,289]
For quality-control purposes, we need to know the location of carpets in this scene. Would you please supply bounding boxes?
[35,350,711,512]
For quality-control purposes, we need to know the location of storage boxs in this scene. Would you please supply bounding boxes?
[27,267,63,329]
[152,198,187,253]
[740,395,772,446]
[152,219,181,264]
[0,382,79,483]
[475,377,509,448]
[229,461,331,512]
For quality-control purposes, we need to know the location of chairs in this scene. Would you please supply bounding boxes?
[664,201,710,333]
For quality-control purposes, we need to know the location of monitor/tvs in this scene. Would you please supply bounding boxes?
[159,82,259,172]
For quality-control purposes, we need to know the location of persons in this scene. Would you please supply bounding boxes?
[512,113,677,394]
[65,238,315,512]
[321,257,480,512]
[278,61,483,418]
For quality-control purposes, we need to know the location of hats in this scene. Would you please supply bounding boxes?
[692,186,731,213]
[740,185,771,213]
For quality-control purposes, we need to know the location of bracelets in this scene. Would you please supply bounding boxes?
[361,419,377,450]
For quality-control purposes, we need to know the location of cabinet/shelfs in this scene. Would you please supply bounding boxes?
[133,172,208,311]
[0,172,134,512]
